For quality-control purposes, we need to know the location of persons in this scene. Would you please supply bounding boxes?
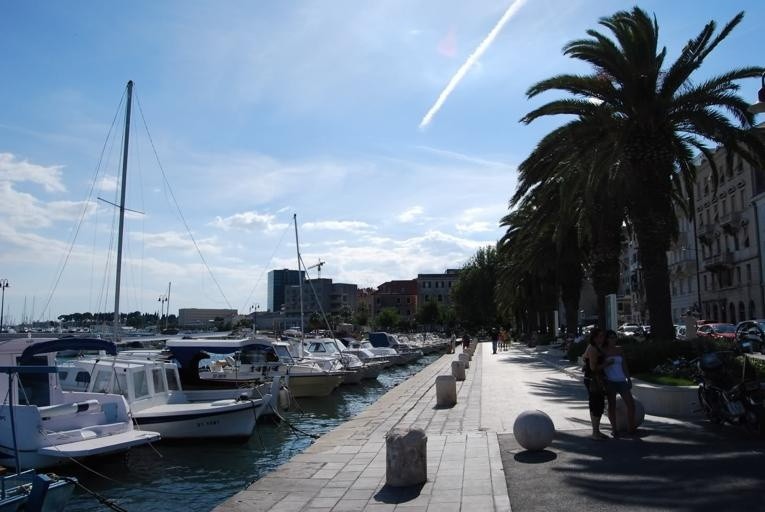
[603,328,645,438]
[489,323,511,353]
[462,332,469,350]
[580,327,603,441]
[684,310,697,339]
[450,330,456,354]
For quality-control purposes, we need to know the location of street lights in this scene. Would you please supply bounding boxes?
[679,245,704,320]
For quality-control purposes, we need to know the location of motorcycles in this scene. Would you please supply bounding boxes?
[680,340,765,438]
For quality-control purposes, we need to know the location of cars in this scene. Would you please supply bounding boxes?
[540,316,764,356]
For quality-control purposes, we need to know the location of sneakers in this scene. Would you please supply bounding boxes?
[593,427,645,441]
